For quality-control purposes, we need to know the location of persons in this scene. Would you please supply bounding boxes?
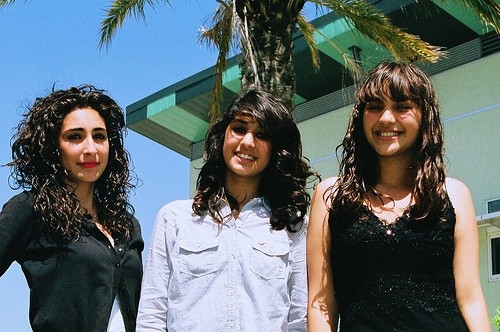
[137,87,307,331]
[1,82,143,332]
[306,59,491,332]
